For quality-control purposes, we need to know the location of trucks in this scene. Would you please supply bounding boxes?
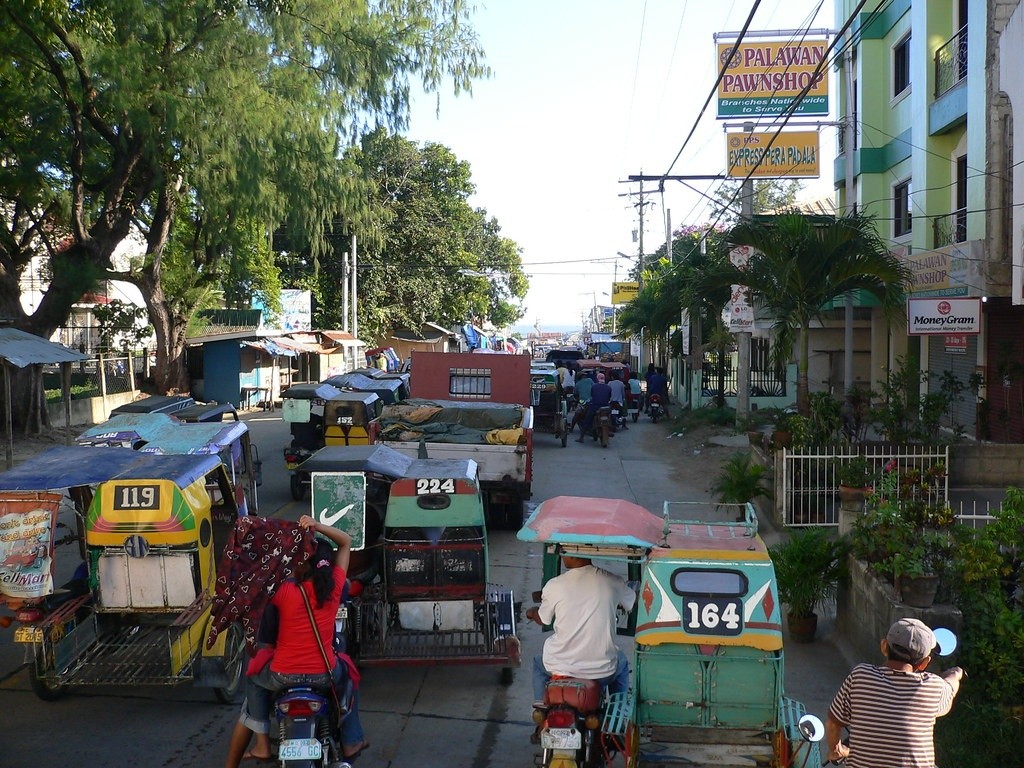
[367,348,535,531]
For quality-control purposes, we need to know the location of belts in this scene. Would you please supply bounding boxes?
[275,671,326,683]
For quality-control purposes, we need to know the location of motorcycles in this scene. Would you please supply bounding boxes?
[516,502,819,768]
[0,393,260,703]
[530,357,661,449]
[283,366,412,502]
[293,441,520,687]
[267,684,352,768]
[798,625,970,767]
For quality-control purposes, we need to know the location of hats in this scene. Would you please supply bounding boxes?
[887,618,937,664]
[597,373,605,384]
[577,371,588,377]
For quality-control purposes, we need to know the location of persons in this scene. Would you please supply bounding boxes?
[224,515,369,768]
[829,616,963,768]
[549,357,670,447]
[290,404,323,450]
[526,557,638,752]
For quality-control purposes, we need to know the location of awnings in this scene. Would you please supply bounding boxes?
[243,330,368,356]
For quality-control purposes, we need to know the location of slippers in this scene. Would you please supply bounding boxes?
[242,750,273,761]
[342,740,371,762]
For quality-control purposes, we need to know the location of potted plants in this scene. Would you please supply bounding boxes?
[893,460,958,608]
[764,403,796,448]
[709,452,777,521]
[839,453,872,501]
[766,525,855,642]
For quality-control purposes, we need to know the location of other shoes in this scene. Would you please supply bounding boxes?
[575,440,584,444]
[622,426,629,430]
[644,409,649,414]
[530,725,541,744]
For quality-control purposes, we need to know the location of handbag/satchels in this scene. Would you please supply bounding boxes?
[331,656,354,728]
